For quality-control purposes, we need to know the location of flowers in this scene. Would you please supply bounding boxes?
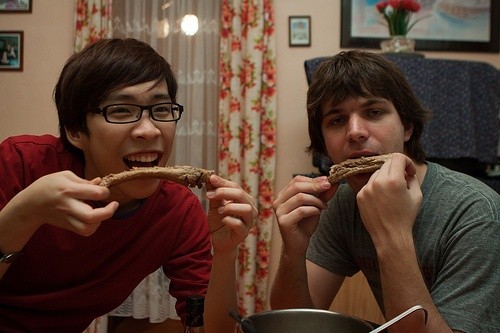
[372,0,433,36]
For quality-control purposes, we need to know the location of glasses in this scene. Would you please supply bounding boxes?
[89,100,185,123]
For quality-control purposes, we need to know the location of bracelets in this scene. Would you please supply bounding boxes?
[0,248,24,264]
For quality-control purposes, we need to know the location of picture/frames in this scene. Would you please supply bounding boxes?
[340,0,500,53]
[0,31,23,72]
[0,0,32,14]
[288,15,312,48]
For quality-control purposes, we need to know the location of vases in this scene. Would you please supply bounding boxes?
[380,36,416,55]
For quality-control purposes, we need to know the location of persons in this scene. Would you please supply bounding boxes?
[268,52,500,333]
[0,37,258,333]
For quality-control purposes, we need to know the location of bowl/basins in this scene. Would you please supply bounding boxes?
[239,309,373,333]
